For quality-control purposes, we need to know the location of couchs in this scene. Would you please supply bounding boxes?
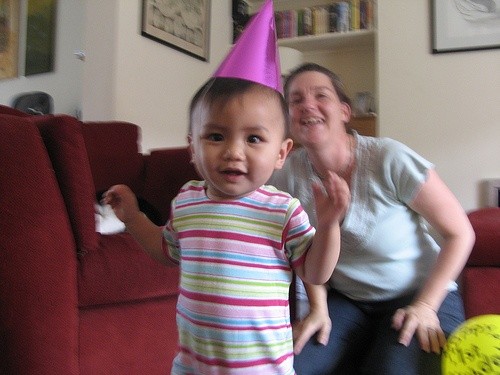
[0,105,203,375]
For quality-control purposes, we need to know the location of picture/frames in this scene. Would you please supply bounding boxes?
[143,1,211,64]
[430,1,499,54]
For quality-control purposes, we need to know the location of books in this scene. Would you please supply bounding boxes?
[232,0,374,45]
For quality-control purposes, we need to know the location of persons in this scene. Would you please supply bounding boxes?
[103,0,350,375]
[263,61,477,375]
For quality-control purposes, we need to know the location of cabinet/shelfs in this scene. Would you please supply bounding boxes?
[260,3,381,139]
